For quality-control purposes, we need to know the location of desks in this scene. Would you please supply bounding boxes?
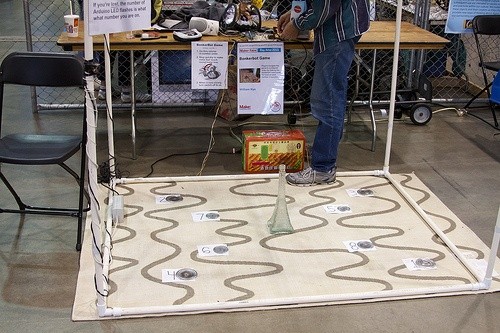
[56,20,451,160]
[376,1,469,98]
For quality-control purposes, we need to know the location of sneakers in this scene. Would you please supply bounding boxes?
[286,166,336,186]
[304,145,312,161]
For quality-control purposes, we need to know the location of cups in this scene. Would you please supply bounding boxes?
[64,15,79,38]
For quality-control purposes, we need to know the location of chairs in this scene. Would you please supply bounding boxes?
[0,52,90,254]
[464,15,500,131]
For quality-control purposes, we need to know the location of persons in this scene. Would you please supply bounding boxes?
[277,0,371,187]
[79,50,151,102]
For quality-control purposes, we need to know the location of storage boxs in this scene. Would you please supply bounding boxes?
[242,130,306,174]
